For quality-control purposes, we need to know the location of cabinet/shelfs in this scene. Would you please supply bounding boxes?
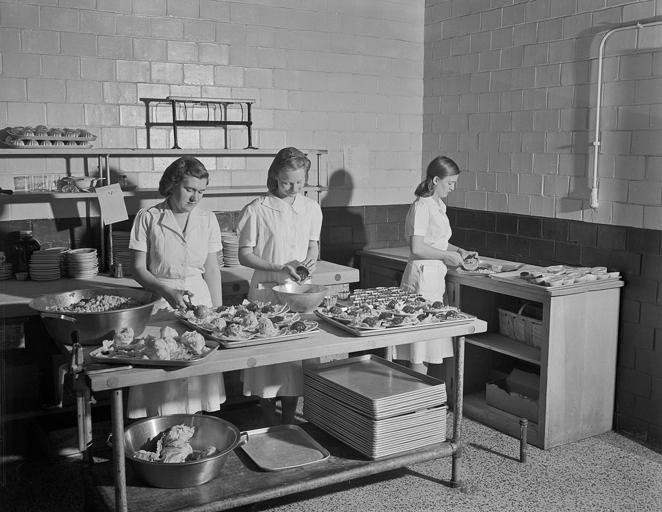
[365,266,455,386]
[0,148,329,199]
[460,283,545,440]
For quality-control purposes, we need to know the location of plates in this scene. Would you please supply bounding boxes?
[0,263,12,281]
[30,248,99,282]
[105,230,134,277]
[218,232,241,268]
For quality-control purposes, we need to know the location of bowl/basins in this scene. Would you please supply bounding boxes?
[272,282,329,314]
[27,288,161,345]
[124,413,241,489]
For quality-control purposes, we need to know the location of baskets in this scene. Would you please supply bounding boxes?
[496,300,544,349]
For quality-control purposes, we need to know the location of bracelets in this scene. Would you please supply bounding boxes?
[457,247,463,257]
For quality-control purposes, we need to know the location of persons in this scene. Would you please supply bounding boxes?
[125,153,228,420]
[389,154,479,376]
[234,146,324,427]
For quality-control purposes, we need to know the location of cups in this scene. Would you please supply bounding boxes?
[13,175,59,192]
